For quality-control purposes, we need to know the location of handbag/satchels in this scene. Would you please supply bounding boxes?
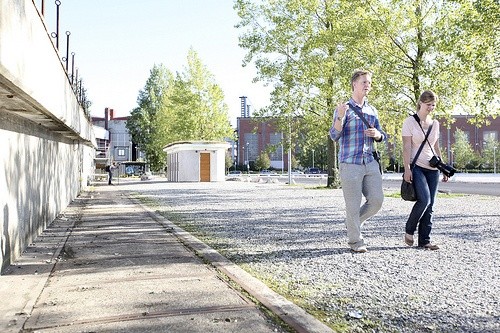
[401,174,416,201]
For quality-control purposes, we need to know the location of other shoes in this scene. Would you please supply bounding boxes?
[351,243,367,252]
[405,232,413,246]
[424,243,438,250]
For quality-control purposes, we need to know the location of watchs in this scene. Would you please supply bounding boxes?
[338,117,343,121]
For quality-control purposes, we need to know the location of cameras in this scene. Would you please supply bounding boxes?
[429,155,457,178]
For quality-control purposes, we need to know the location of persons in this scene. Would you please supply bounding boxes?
[330,70,388,252]
[402,90,449,250]
[108,162,118,184]
